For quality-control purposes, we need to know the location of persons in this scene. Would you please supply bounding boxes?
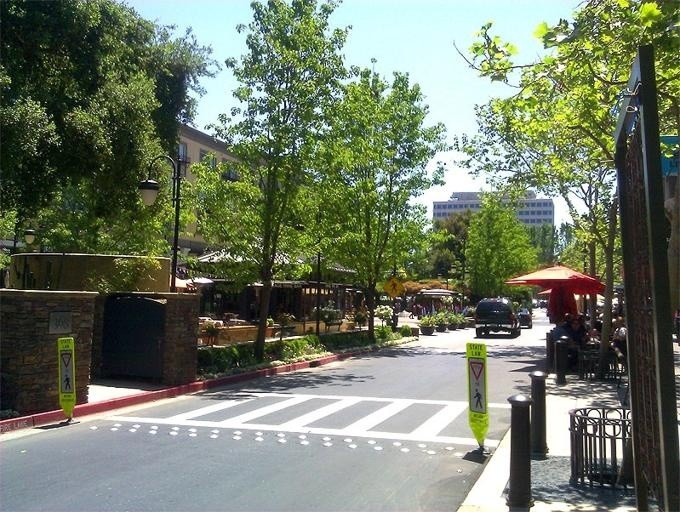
[408,298,461,320]
[553,313,628,375]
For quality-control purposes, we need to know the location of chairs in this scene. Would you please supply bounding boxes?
[324,309,343,333]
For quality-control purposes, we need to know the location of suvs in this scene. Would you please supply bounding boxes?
[475,297,520,338]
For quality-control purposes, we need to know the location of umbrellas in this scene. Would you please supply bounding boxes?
[504,262,606,326]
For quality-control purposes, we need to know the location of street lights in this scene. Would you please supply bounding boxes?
[140,155,182,293]
[14,219,38,253]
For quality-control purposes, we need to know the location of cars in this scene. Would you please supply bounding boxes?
[517,307,533,328]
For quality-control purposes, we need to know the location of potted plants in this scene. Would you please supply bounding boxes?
[418,310,468,335]
